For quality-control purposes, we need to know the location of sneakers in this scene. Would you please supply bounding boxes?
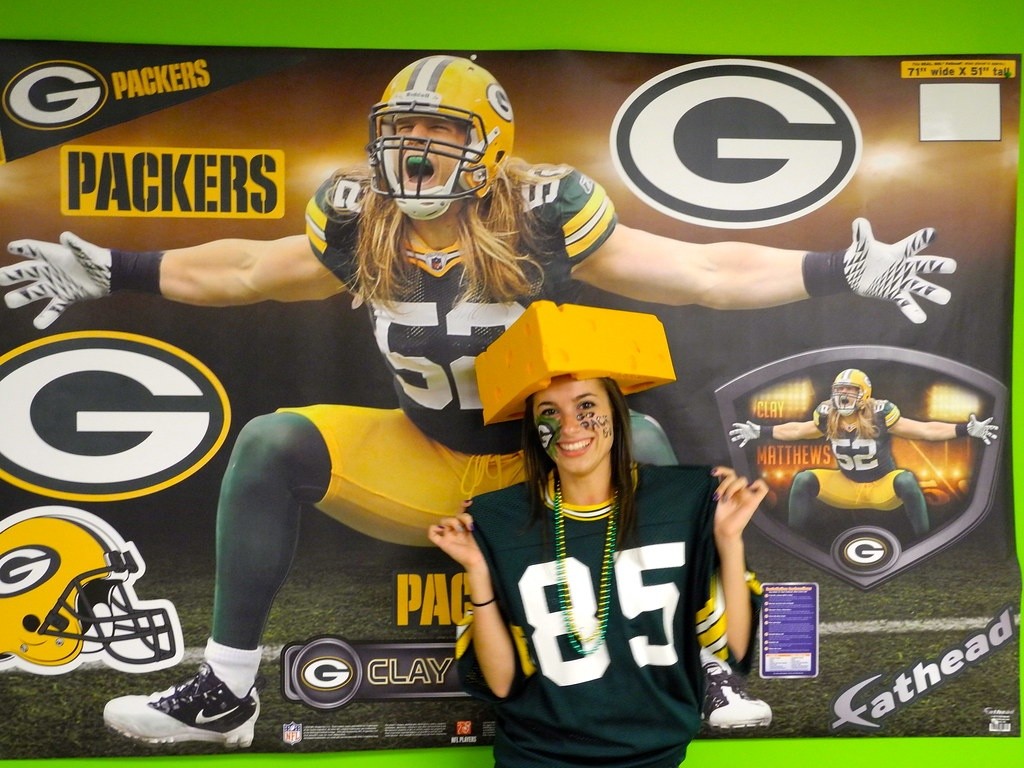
[103,661,266,747]
[700,660,773,728]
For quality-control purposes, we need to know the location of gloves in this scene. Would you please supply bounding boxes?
[0,231,162,330]
[802,216,957,324]
[728,421,774,448]
[955,413,999,445]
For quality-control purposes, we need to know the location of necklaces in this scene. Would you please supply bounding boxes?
[553,476,622,657]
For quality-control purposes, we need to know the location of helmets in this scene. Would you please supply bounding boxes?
[831,368,871,413]
[375,55,514,220]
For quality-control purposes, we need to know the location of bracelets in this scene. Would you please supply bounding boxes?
[469,597,496,607]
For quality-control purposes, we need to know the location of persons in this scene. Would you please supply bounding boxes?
[729,367,998,538]
[426,375,768,767]
[0,55,960,749]
